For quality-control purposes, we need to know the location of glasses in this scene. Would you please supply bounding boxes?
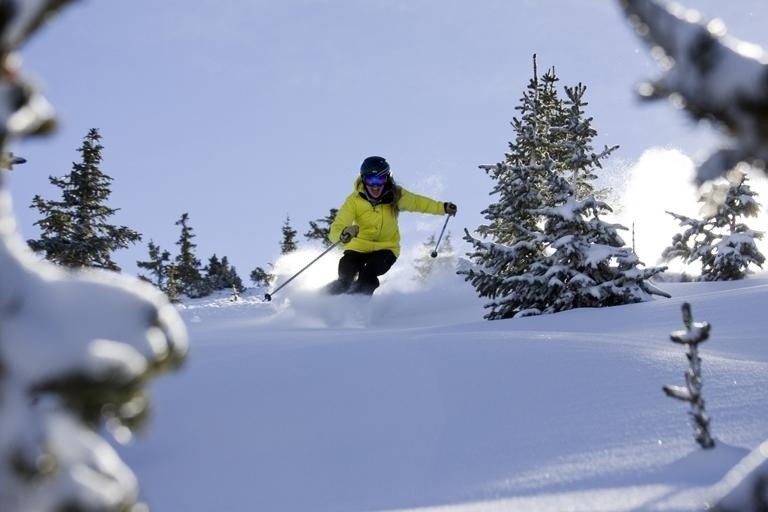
[361,176,387,186]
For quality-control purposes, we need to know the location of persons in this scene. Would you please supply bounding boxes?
[318,155,458,296]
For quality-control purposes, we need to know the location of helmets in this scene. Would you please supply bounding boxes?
[361,156,390,183]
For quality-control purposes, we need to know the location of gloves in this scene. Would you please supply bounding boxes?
[445,202,457,216]
[343,225,359,238]
